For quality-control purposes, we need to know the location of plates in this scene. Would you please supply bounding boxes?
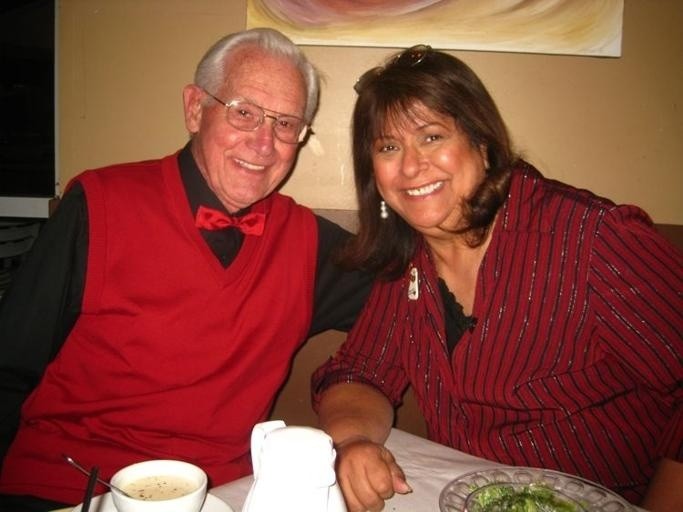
[437,465,638,512]
[71,491,234,512]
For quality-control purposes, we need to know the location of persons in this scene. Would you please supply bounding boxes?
[0,27,377,511]
[309,45,682,512]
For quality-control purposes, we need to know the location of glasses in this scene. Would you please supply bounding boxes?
[354,44,430,94]
[201,87,308,145]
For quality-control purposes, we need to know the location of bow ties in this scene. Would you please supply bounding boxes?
[191,205,268,236]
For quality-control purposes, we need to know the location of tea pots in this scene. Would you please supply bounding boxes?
[236,418,354,512]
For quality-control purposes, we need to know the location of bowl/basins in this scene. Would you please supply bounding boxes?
[107,458,209,512]
